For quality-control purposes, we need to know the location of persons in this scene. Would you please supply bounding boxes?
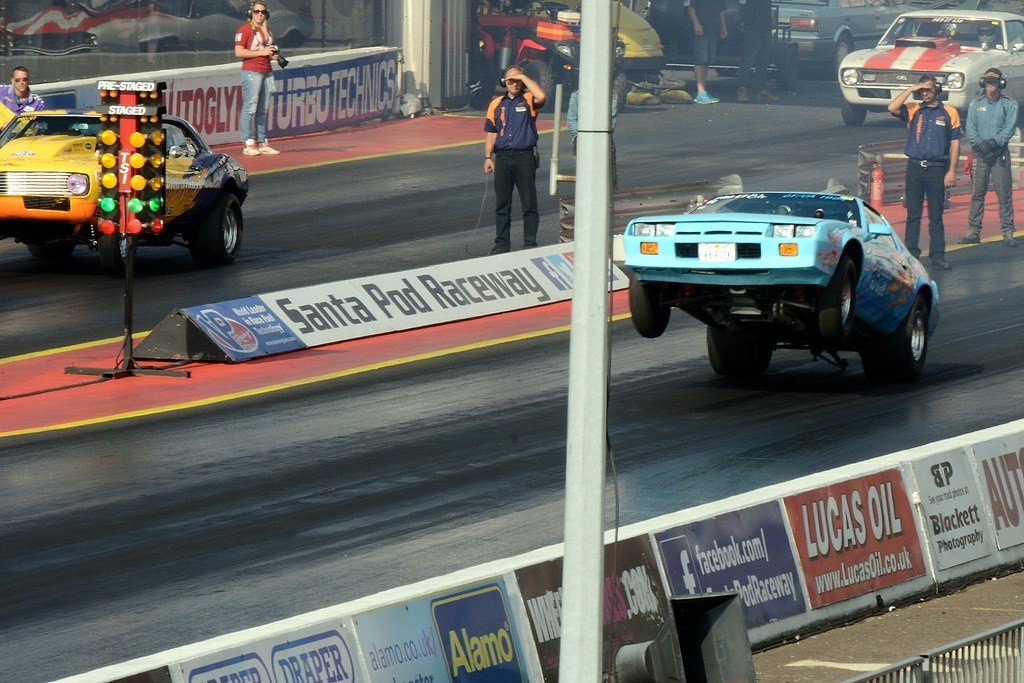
[234,1,280,155]
[736,0,779,102]
[566,83,620,189]
[957,68,1019,247]
[0,66,48,131]
[484,65,546,256]
[889,76,964,270]
[683,0,728,104]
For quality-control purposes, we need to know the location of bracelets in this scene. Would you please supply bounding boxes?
[485,157,491,159]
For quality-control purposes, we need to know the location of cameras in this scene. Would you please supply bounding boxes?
[271,44,289,69]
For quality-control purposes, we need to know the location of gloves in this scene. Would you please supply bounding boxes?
[978,143,996,165]
[976,139,999,156]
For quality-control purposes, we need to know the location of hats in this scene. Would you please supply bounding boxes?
[984,68,1001,83]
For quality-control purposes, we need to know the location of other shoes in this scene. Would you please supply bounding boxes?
[737,87,749,102]
[756,90,779,103]
[491,248,506,254]
[934,259,951,270]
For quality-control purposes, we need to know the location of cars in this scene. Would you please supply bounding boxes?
[624,193,940,385]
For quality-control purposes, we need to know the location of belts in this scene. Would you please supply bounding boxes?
[909,156,945,169]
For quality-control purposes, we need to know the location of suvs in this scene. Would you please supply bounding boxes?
[0,109,248,274]
[472,0,664,116]
[773,0,1024,139]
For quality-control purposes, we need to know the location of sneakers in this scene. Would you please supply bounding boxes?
[1003,230,1016,247]
[957,228,980,245]
[243,139,261,156]
[694,91,720,104]
[257,138,280,155]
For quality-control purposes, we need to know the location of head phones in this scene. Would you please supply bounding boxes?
[500,65,527,88]
[979,77,1006,89]
[916,75,942,96]
[247,2,269,20]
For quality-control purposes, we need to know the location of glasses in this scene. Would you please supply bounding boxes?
[253,9,266,14]
[12,78,29,82]
